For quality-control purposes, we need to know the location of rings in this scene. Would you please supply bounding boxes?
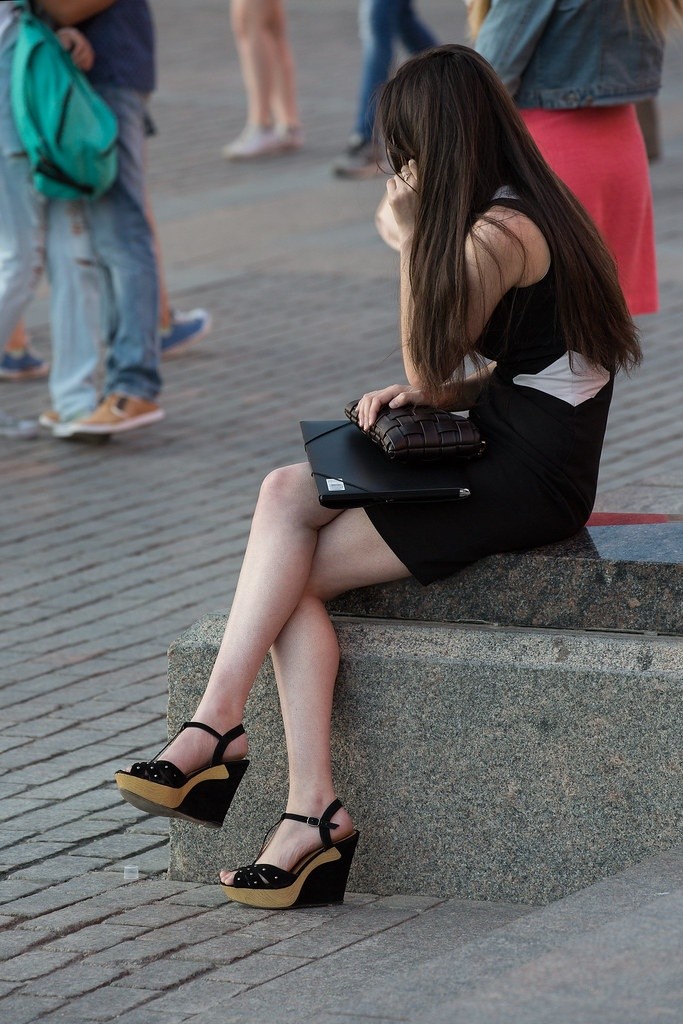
[400,172,411,180]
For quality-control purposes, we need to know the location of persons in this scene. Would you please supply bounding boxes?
[330,0,443,176]
[463,0,683,316]
[114,43,644,909]
[0,0,211,439]
[221,0,302,159]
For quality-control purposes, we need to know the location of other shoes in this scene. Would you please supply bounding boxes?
[332,139,393,177]
[221,126,278,162]
[270,123,307,154]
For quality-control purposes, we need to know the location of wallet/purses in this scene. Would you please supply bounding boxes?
[343,397,487,458]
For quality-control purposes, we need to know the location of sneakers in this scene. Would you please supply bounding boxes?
[154,307,211,357]
[43,392,166,438]
[0,352,50,380]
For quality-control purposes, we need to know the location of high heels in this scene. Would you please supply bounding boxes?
[113,721,250,829]
[216,797,359,910]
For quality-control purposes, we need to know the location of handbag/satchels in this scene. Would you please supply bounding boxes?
[300,419,473,510]
[8,0,119,202]
